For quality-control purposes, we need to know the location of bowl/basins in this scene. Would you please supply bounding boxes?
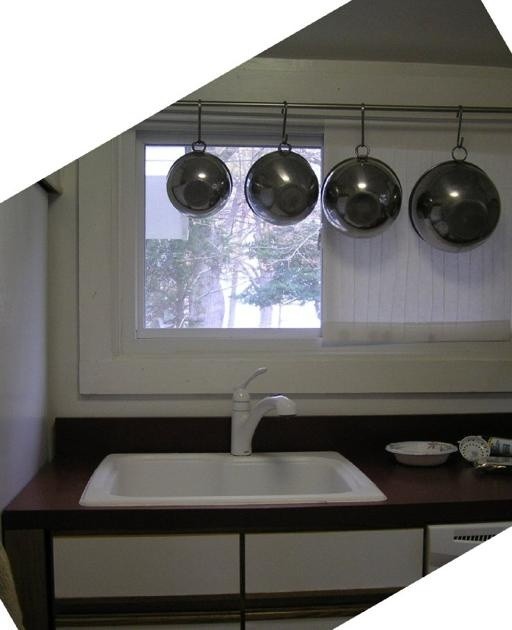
[387,441,458,466]
[457,436,490,463]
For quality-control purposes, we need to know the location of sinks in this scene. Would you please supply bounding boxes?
[78,451,388,505]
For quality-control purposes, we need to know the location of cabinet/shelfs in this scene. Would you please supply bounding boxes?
[428,501,510,574]
[0,505,426,630]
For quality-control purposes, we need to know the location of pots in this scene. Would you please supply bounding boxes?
[162,151,232,215]
[244,151,318,225]
[408,159,501,252]
[321,158,403,235]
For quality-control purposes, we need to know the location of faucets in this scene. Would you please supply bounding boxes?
[231,368,297,456]
